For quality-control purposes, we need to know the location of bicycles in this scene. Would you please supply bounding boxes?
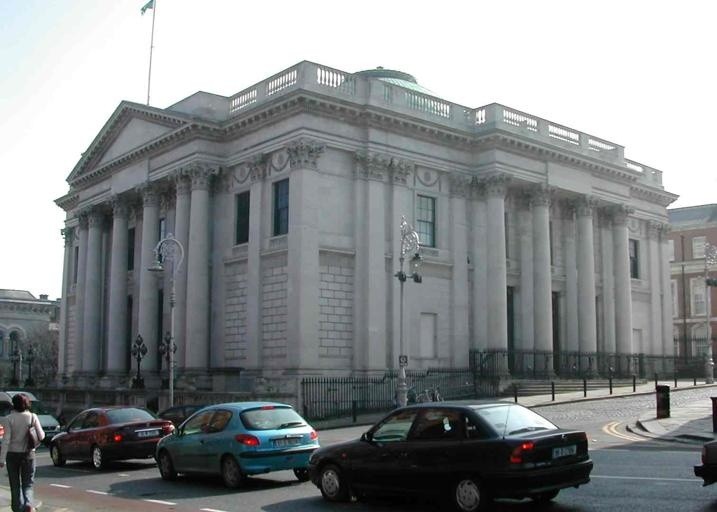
[410,385,445,402]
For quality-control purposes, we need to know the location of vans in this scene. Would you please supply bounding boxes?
[0,393,14,444]
[6,391,59,447]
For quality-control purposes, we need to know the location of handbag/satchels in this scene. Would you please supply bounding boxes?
[28,426,41,448]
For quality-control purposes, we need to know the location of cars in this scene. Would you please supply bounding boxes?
[152,400,320,487]
[306,396,592,510]
[155,405,208,427]
[693,440,717,487]
[48,403,175,471]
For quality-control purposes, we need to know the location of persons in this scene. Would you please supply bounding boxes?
[0,392,46,512]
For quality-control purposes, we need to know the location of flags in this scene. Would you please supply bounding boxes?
[140,0,154,14]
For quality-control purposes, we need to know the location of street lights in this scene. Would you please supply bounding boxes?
[24,343,36,388]
[395,215,423,410]
[131,334,148,389]
[159,333,179,390]
[149,230,185,408]
[700,243,716,385]
[7,344,22,386]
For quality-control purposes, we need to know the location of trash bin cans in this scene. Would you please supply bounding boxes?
[710,397,717,433]
